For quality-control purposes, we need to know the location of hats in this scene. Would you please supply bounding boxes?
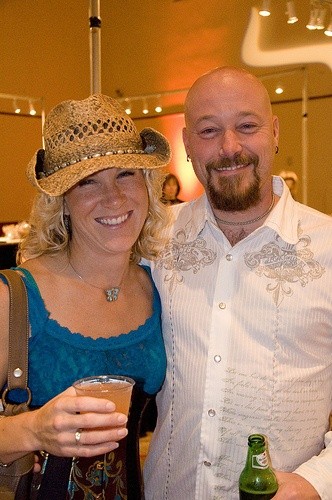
[25,95,173,197]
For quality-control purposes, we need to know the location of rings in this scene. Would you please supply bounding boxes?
[73,427,86,445]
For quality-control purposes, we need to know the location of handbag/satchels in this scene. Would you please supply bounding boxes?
[1,270,33,500]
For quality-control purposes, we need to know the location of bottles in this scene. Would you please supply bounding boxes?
[239,434,278,500]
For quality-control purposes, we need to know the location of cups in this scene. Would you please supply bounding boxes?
[72,375,135,433]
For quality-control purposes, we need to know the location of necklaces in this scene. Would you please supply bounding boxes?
[59,241,140,303]
[210,192,277,225]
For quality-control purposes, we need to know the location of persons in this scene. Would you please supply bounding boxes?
[132,64,331,499]
[0,92,175,500]
[158,173,186,206]
